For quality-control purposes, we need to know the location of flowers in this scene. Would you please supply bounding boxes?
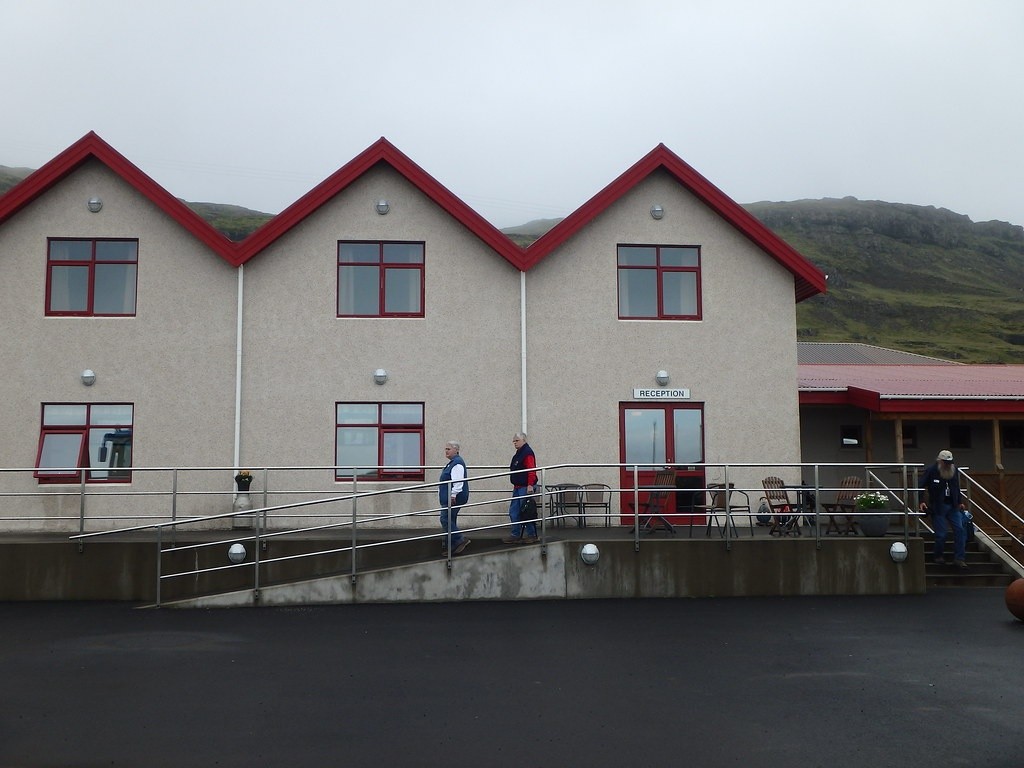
[853,492,890,512]
[234,471,253,483]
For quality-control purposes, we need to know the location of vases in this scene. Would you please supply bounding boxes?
[859,509,891,538]
[238,481,250,491]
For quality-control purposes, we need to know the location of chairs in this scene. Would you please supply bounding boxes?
[578,483,612,528]
[820,475,862,536]
[627,470,677,536]
[532,486,554,529]
[780,485,824,537]
[551,483,584,528]
[706,483,754,539]
[689,483,735,539]
[762,476,807,538]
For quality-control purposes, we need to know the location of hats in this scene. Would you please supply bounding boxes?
[938,449,954,465]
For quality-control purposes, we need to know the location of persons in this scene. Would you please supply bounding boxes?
[918,450,968,569]
[502,432,539,543]
[438,441,471,556]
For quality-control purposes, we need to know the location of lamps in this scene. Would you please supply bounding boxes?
[655,369,672,387]
[80,369,96,386]
[375,200,390,215]
[650,202,665,220]
[86,195,103,213]
[373,368,388,385]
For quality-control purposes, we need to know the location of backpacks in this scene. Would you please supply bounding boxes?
[519,495,538,540]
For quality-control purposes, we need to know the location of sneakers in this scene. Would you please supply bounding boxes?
[934,556,945,564]
[953,558,968,569]
[522,536,542,544]
[453,536,471,555]
[502,534,527,544]
[440,548,455,557]
